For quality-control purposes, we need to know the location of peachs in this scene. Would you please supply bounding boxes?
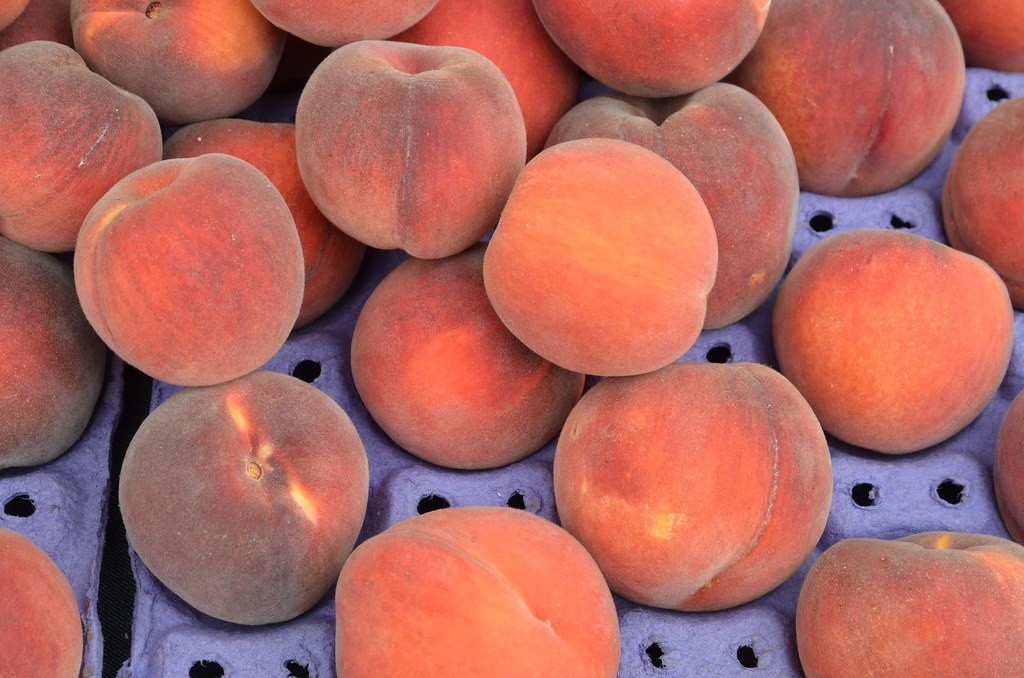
[0,0,1024,678]
